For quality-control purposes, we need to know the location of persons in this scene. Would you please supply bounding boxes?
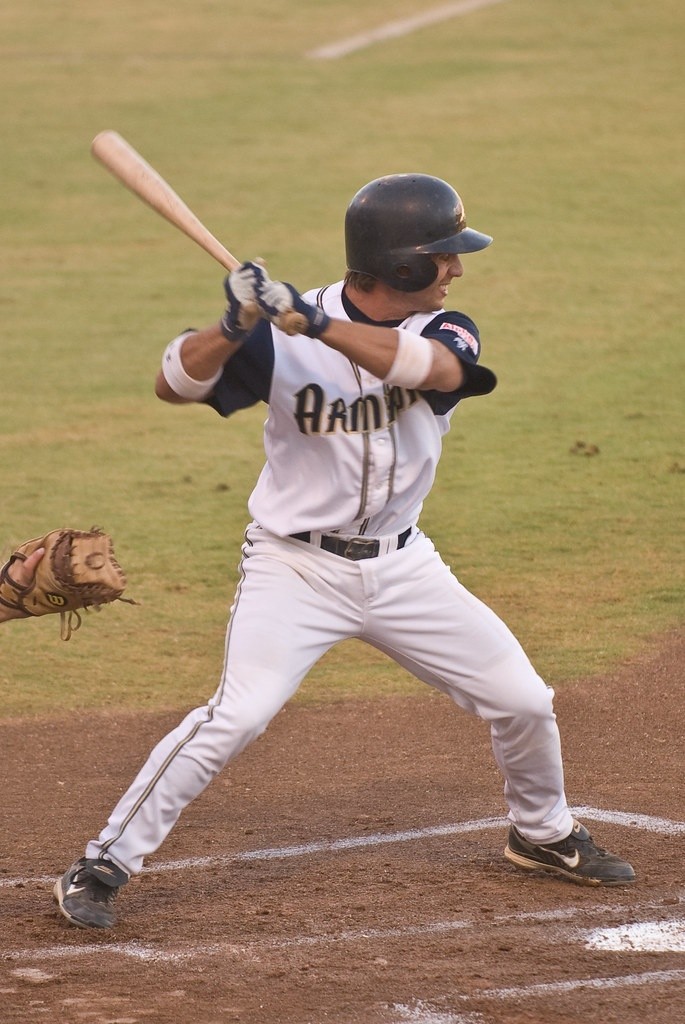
[0,547,46,623]
[53,174,636,931]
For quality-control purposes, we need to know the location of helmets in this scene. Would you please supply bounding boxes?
[345,173,493,292]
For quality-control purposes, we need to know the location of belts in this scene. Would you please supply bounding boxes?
[290,527,415,560]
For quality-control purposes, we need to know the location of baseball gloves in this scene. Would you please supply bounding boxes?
[0,523,135,623]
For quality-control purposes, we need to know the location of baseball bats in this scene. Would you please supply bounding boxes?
[86,124,313,342]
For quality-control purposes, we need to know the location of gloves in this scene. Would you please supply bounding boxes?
[256,278,329,338]
[220,256,271,342]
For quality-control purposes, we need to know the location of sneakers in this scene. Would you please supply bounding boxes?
[504,823,636,887]
[52,856,129,929]
